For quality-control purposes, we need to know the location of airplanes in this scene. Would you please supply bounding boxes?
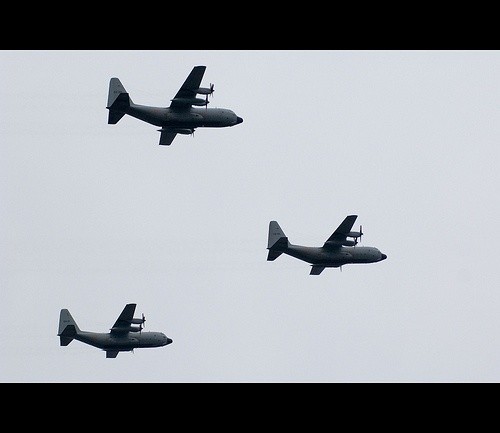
[105,65,244,146]
[57,303,173,359]
[267,215,387,275]
